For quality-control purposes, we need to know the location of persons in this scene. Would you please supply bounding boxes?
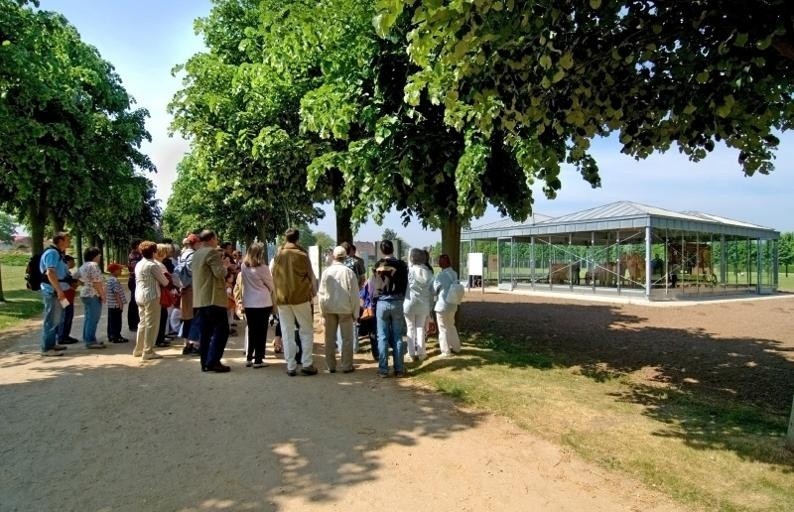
[319,243,461,377]
[56,255,79,345]
[70,245,109,350]
[38,232,72,358]
[105,262,129,344]
[129,227,317,377]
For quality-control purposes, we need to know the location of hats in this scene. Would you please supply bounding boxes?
[107,265,122,270]
[333,246,347,257]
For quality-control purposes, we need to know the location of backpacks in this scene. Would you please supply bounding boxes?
[171,253,194,287]
[25,244,72,291]
[443,271,464,305]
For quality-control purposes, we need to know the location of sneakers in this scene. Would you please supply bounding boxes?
[201,342,461,378]
[229,328,237,336]
[42,326,199,359]
[231,324,237,327]
[234,315,240,320]
